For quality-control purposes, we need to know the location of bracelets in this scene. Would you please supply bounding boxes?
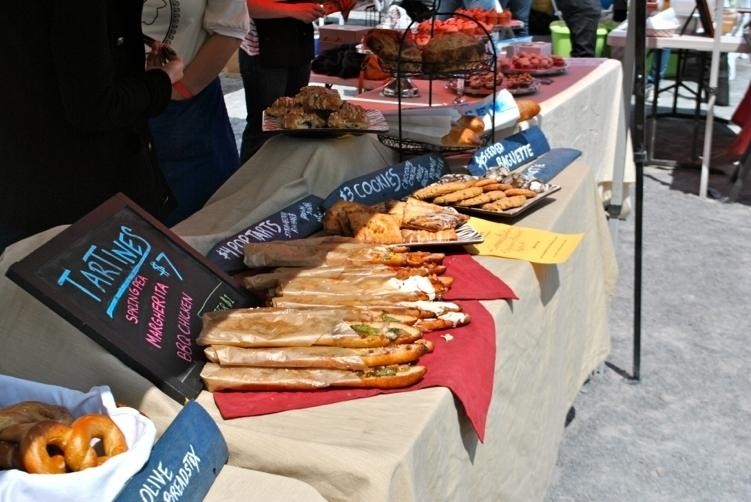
[174,82,192,101]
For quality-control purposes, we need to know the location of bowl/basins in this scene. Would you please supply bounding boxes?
[645,23,679,37]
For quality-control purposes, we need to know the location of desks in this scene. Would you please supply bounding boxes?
[308,44,632,221]
[605,1,744,200]
[0,137,619,502]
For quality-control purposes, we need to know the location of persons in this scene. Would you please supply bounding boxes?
[140,0,252,228]
[0,0,184,251]
[238,0,328,167]
[428,0,672,103]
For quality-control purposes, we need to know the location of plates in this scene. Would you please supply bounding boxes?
[262,109,390,137]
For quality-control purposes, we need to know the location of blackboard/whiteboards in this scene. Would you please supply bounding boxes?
[4,191,267,406]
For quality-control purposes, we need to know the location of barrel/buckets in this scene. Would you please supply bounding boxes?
[550,20,607,58]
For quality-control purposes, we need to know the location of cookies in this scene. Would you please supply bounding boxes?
[323,177,537,243]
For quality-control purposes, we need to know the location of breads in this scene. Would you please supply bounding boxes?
[344,246,470,389]
[265,86,369,130]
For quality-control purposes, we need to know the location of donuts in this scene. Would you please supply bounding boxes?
[0,400,127,474]
